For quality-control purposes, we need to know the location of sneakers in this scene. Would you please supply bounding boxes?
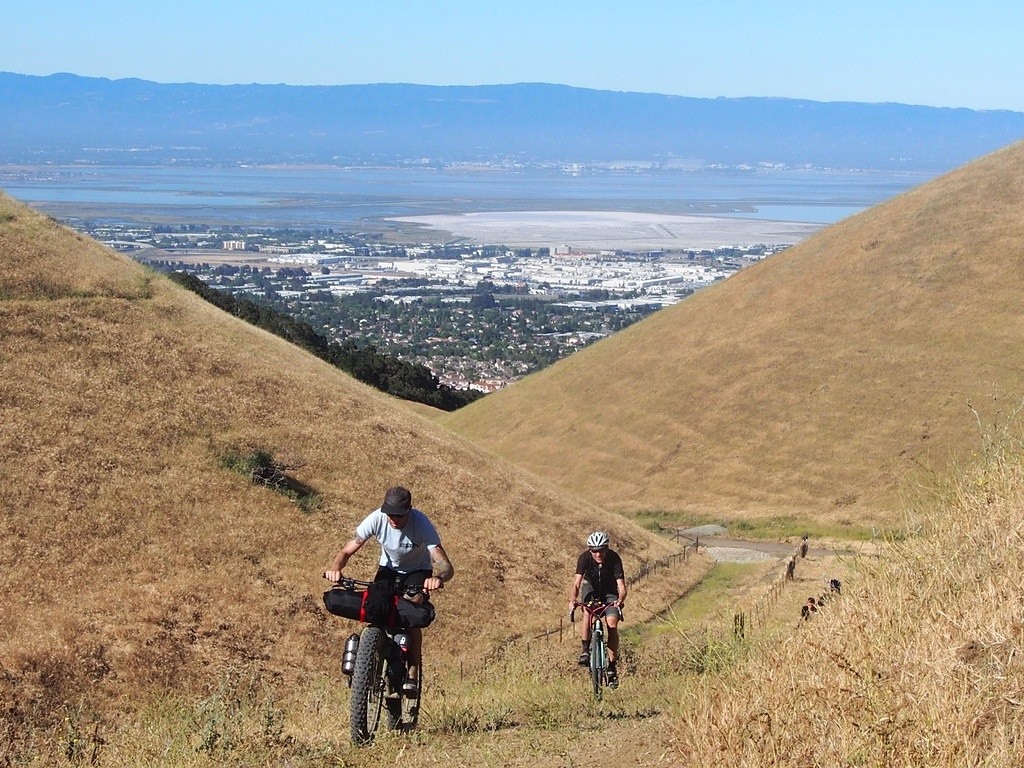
[578,651,590,667]
[607,668,619,689]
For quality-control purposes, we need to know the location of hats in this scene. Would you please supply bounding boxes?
[381,487,412,515]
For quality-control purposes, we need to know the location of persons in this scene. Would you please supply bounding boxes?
[796,579,841,628]
[326,487,455,697]
[569,531,627,689]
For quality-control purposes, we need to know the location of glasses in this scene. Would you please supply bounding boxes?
[387,510,411,518]
[590,549,605,553]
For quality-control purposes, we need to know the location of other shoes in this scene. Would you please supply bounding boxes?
[403,680,417,700]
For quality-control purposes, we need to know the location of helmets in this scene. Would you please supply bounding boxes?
[587,531,609,551]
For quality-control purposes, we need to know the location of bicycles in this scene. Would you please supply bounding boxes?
[323,572,444,748]
[570,600,624,701]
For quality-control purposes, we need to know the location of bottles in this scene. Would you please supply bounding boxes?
[343,633,360,673]
